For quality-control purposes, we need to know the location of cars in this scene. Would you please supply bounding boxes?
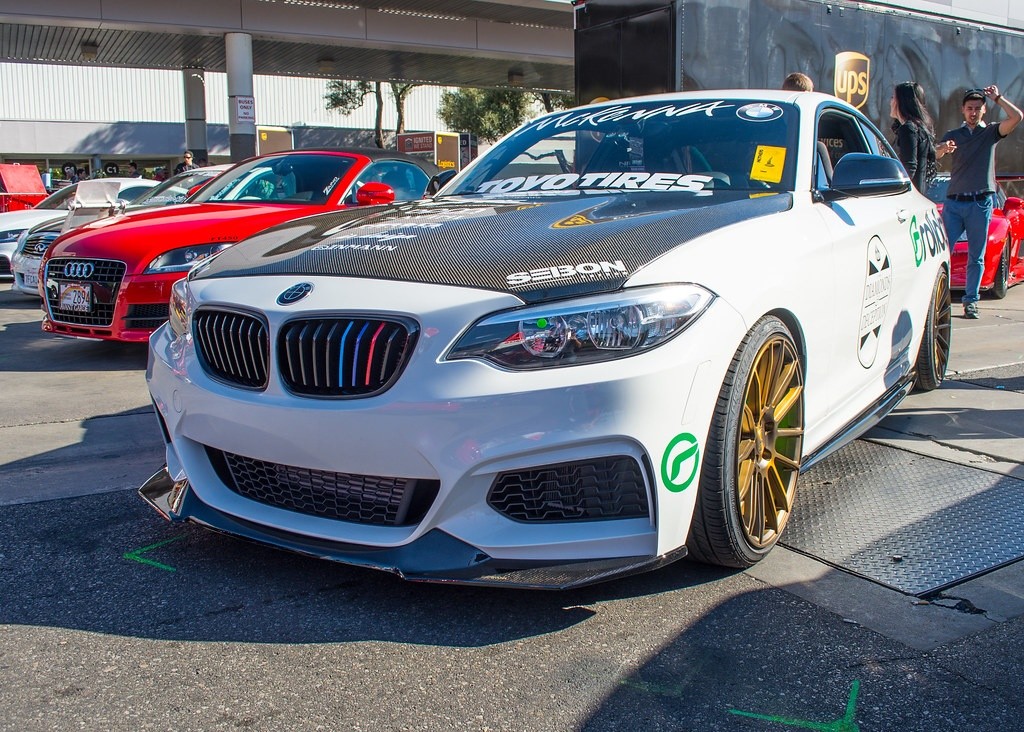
[0,169,1023,315]
[34,146,446,345]
[134,87,954,595]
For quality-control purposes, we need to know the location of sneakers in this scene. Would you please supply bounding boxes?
[965,302,980,318]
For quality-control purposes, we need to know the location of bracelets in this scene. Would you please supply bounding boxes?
[994,94,1001,103]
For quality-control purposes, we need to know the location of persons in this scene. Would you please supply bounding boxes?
[174,151,199,175]
[198,158,216,167]
[783,72,813,91]
[935,85,1024,319]
[890,82,936,196]
[128,163,140,178]
[72,169,86,183]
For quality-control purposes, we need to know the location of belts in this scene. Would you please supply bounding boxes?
[949,194,991,202]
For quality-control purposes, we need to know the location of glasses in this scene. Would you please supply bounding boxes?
[964,88,986,100]
[184,156,192,159]
